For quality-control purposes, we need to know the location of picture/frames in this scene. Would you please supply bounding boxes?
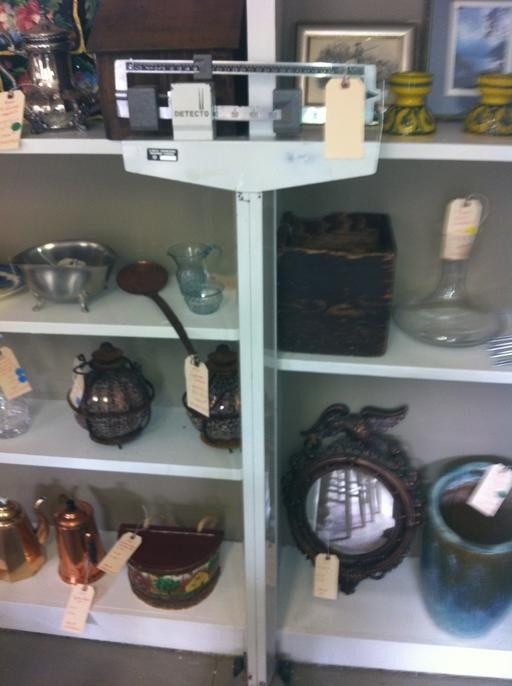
[423,1,512,122]
[294,21,416,126]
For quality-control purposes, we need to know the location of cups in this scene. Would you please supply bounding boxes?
[166,240,215,297]
[180,276,225,318]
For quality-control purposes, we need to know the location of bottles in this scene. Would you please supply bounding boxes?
[18,3,94,135]
[462,70,512,140]
[379,68,439,136]
[1,335,35,442]
[393,192,507,350]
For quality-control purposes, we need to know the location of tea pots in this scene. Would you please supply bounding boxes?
[0,492,50,584]
[53,498,111,586]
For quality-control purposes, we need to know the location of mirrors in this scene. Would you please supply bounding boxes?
[281,402,423,596]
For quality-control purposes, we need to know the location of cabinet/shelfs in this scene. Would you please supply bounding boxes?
[2,123,257,686]
[257,122,512,686]
